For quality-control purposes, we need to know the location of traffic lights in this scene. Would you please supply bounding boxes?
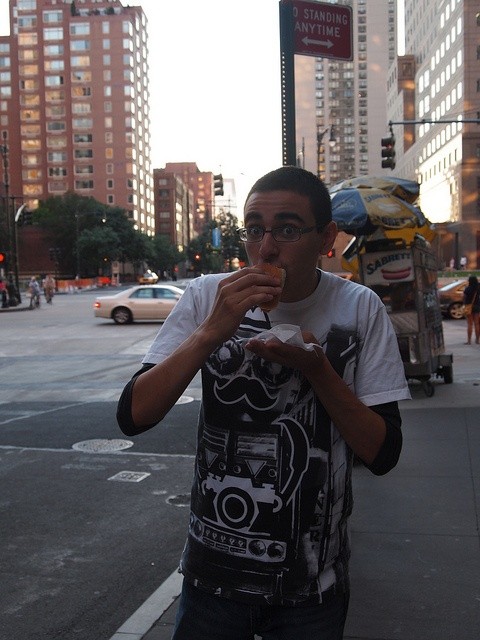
[382,137,396,169]
[194,253,201,261]
[213,173,223,197]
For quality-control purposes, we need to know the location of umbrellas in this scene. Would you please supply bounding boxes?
[329,175,420,205]
[342,218,435,275]
[331,188,427,285]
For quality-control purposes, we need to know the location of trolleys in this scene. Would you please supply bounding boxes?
[360,241,455,397]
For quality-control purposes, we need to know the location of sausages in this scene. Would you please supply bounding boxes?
[381,266,412,279]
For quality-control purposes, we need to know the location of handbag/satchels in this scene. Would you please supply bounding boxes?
[462,304,473,316]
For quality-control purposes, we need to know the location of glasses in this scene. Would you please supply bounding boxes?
[237,224,319,242]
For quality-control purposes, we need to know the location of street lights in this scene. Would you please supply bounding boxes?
[14,201,27,303]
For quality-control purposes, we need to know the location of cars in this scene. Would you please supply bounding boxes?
[439,280,469,319]
[94,284,185,324]
[139,272,158,284]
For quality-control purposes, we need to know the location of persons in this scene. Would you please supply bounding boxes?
[45,274,56,303]
[28,277,40,309]
[463,277,480,345]
[116,166,412,640]
[450,257,454,268]
[460,255,466,270]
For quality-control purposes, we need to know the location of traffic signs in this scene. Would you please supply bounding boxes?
[291,0,354,61]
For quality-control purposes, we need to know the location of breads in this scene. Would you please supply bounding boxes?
[250,263,286,313]
